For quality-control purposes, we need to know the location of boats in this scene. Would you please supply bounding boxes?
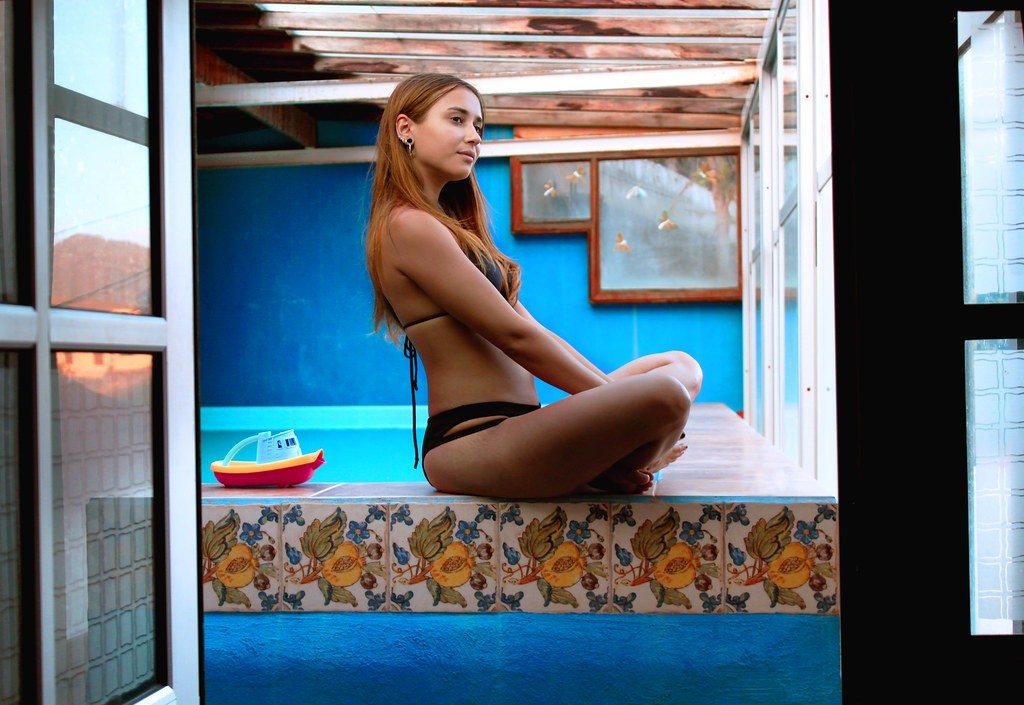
[210,429,326,489]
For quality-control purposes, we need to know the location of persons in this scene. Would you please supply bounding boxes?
[366,73,703,500]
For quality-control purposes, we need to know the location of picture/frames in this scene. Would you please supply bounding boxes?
[508,141,742,304]
